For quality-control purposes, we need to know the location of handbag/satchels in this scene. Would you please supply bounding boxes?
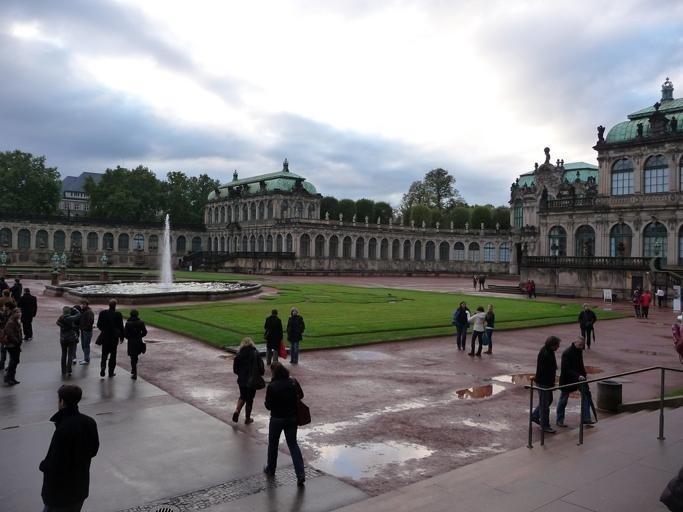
[140,338,147,355]
[481,329,490,345]
[293,399,311,428]
[253,375,265,391]
[95,332,104,346]
[277,342,288,360]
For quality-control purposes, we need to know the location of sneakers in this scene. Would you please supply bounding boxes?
[468,350,492,358]
[72,358,89,365]
[232,410,239,423]
[131,368,137,380]
[542,426,556,434]
[557,420,568,428]
[581,419,595,425]
[297,477,306,488]
[263,464,275,477]
[244,418,254,424]
[531,416,541,426]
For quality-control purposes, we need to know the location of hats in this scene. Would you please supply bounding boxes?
[58,384,83,405]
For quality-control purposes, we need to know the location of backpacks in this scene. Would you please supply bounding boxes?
[0,328,9,346]
[264,316,279,340]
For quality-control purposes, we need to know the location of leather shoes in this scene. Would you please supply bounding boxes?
[108,372,116,377]
[100,367,105,377]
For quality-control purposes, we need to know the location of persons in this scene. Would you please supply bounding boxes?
[287,309,305,363]
[530,336,561,433]
[265,360,305,486]
[452,274,665,357]
[0,277,147,386]
[232,336,264,424]
[39,385,99,512]
[659,323,683,511]
[263,310,283,365]
[556,335,596,428]
